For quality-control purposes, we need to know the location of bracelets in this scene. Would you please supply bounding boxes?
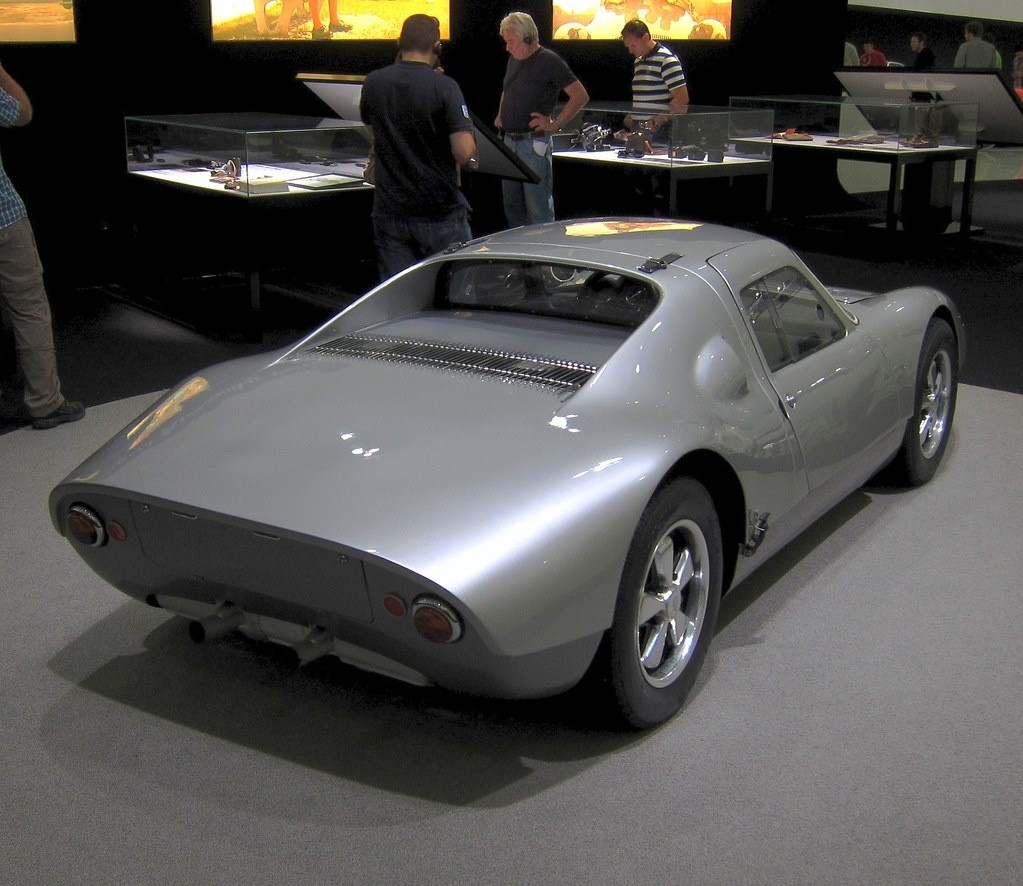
[555,118,564,130]
[652,119,660,130]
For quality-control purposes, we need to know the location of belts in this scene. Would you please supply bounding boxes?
[506,129,546,141]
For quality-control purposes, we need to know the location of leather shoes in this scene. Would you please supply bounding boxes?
[33,399,86,429]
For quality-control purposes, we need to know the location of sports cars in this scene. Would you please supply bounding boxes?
[46,212,968,738]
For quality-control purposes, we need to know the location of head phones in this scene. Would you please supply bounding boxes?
[523,37,532,44]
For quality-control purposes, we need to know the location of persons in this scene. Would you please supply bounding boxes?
[858,41,887,69]
[253,0,299,36]
[297,0,324,19]
[308,0,353,37]
[1,57,89,434]
[620,19,689,145]
[359,12,476,287]
[1011,49,1022,89]
[900,32,945,149]
[952,21,996,69]
[981,33,1003,70]
[494,12,589,227]
